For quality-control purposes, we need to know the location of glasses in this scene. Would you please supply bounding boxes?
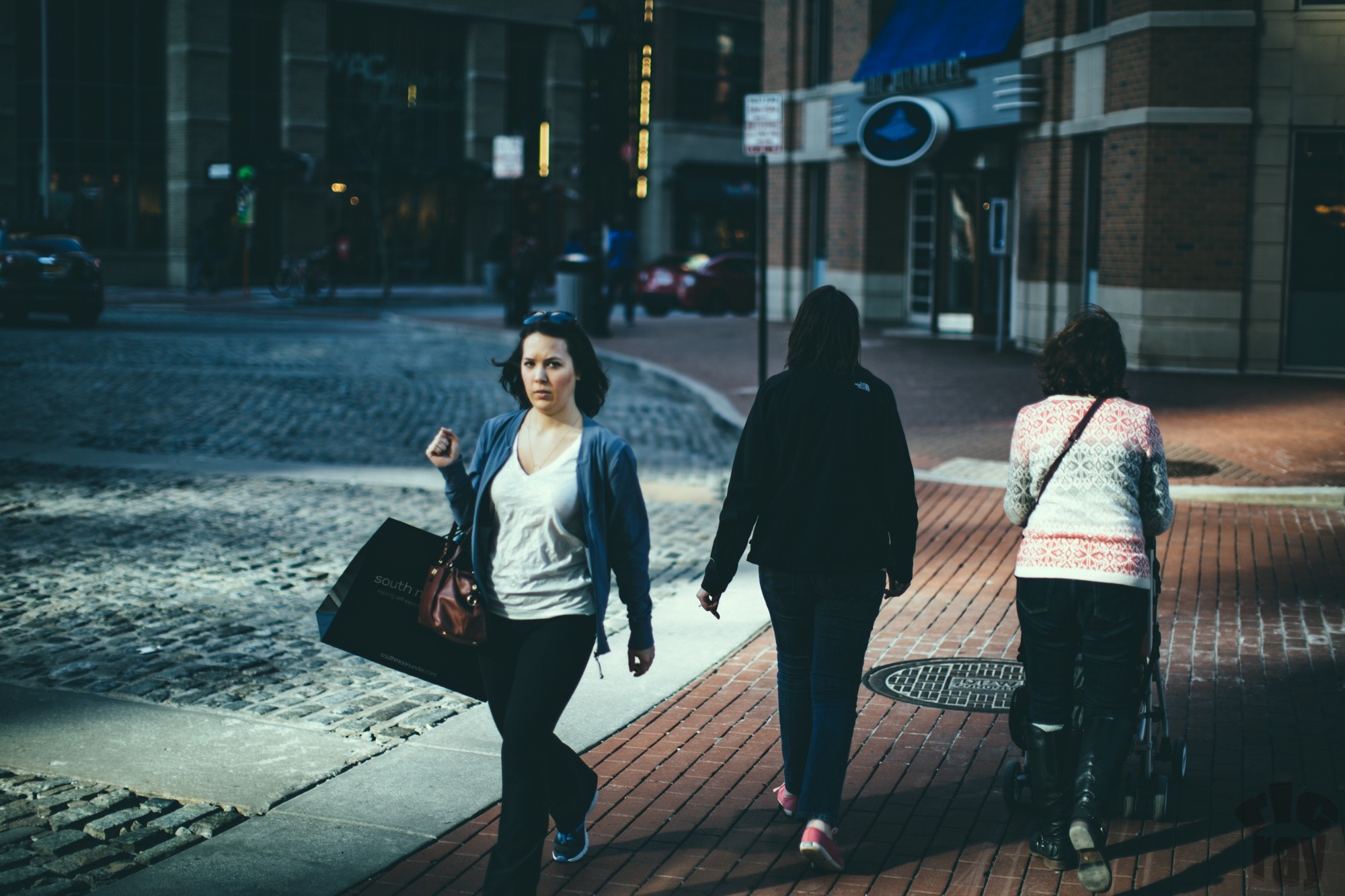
[522,310,580,331]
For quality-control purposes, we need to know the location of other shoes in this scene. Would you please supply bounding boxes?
[1069,818,1113,893]
[1028,823,1072,871]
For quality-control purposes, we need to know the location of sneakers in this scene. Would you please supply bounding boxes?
[552,788,598,863]
[773,781,798,817]
[799,826,846,874]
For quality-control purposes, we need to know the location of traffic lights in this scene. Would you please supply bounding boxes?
[226,161,260,186]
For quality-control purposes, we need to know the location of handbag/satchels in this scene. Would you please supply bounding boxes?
[316,470,493,703]
[416,518,485,645]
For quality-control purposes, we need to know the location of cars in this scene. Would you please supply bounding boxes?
[0,231,106,327]
[633,253,755,318]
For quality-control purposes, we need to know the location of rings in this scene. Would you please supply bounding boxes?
[698,604,702,607]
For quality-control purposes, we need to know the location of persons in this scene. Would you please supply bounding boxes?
[604,215,639,323]
[696,285,919,876]
[425,311,656,896]
[1001,301,1175,896]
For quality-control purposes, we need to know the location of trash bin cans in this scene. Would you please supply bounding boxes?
[555,253,604,338]
